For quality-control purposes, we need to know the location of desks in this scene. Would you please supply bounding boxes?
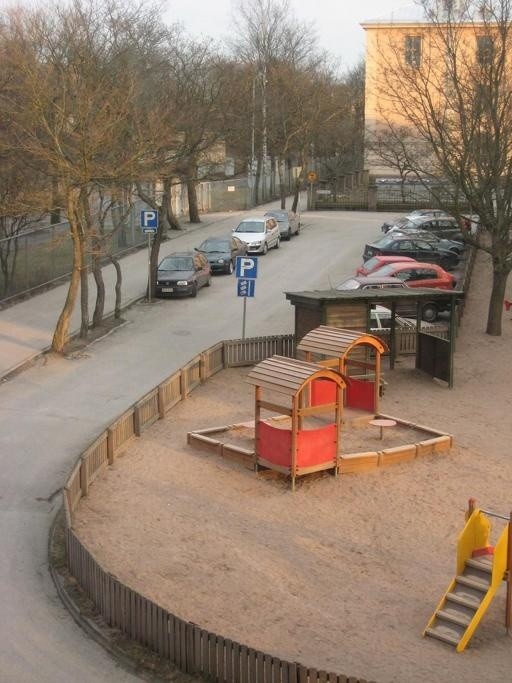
[369,419,397,440]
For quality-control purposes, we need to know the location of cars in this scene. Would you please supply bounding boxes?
[156,210,300,297]
[335,209,471,331]
[316,178,344,200]
